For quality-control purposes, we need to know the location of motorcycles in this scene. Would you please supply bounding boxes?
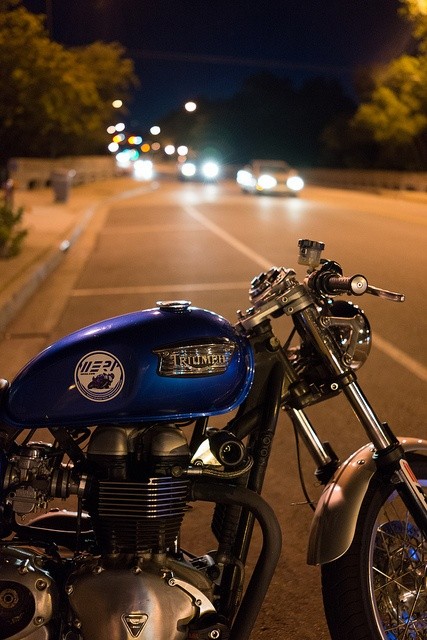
[0,238,427,640]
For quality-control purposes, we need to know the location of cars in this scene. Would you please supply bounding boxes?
[237,158,306,198]
